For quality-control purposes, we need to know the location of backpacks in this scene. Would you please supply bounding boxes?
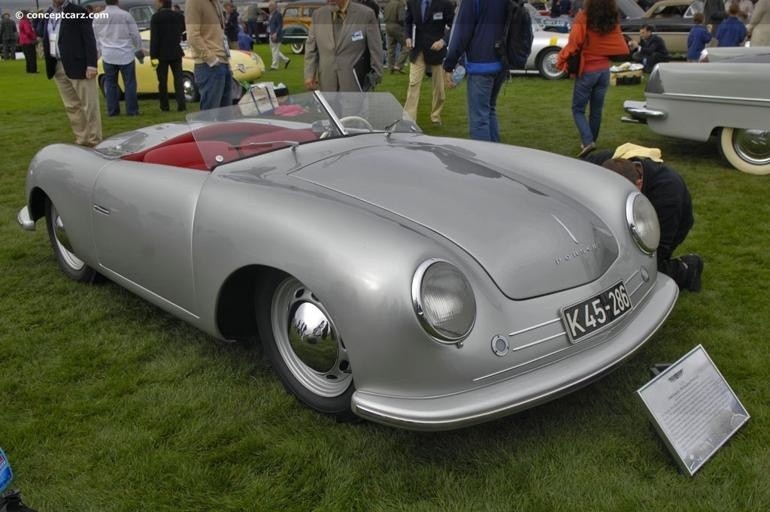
[496,1,533,69]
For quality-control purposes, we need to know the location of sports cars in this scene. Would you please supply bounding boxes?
[18,88,682,435]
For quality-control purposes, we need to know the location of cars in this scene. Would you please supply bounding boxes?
[501,0,715,80]
[619,41,769,176]
[94,32,272,101]
[50,2,389,57]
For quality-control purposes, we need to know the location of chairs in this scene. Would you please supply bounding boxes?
[240,130,319,159]
[142,140,241,172]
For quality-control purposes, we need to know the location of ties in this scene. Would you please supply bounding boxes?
[334,12,342,40]
[424,1,430,19]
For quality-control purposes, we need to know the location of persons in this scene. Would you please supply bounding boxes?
[586,148,706,293]
[398,0,450,124]
[442,0,514,141]
[39,1,103,146]
[557,0,630,159]
[0,0,770,114]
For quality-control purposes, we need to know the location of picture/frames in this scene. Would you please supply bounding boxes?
[633,343,751,477]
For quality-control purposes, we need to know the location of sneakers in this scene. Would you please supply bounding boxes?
[578,142,596,157]
[684,255,703,292]
